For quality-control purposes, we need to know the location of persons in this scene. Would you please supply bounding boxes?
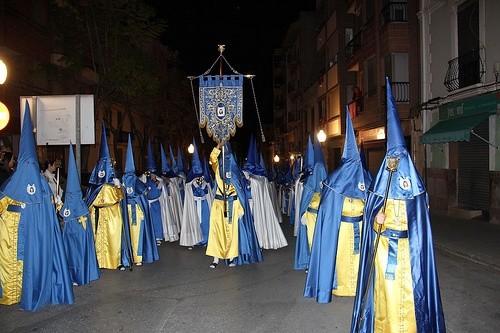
[206,143,263,269]
[0,150,447,333]
[352,87,363,115]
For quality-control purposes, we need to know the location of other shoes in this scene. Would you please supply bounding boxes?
[119,266,126,271]
[209,258,220,268]
[228,258,236,267]
[156,240,161,246]
[188,245,195,250]
[135,262,143,266]
[304,268,309,274]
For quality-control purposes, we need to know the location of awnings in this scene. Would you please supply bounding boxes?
[418,111,495,144]
